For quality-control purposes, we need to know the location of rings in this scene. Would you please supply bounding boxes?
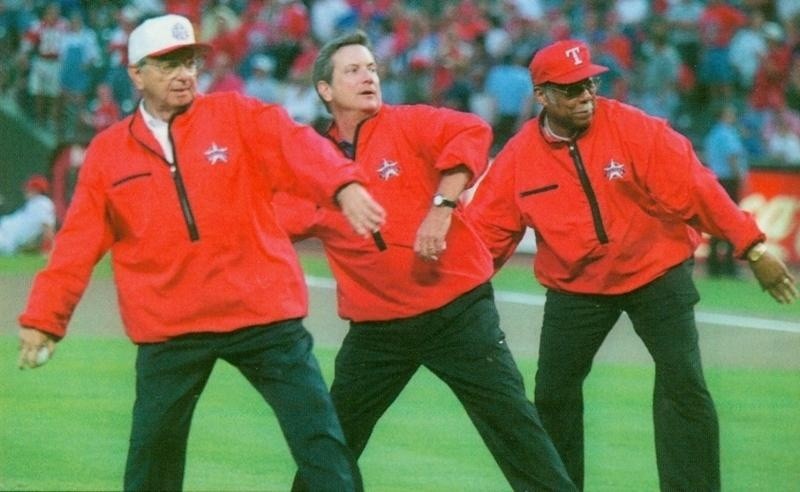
[784,279,789,284]
[427,248,434,251]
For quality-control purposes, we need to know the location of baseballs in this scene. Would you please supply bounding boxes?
[25,347,50,366]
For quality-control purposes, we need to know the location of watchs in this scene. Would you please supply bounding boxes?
[433,194,457,208]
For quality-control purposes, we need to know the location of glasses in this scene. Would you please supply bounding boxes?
[544,78,594,97]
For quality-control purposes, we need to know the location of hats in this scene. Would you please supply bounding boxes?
[528,39,609,87]
[126,13,212,68]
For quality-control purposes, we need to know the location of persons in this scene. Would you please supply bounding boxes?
[170,0,578,154]
[269,30,578,492]
[1,0,161,213]
[0,168,56,261]
[464,34,796,492]
[12,14,385,491]
[573,0,800,279]
